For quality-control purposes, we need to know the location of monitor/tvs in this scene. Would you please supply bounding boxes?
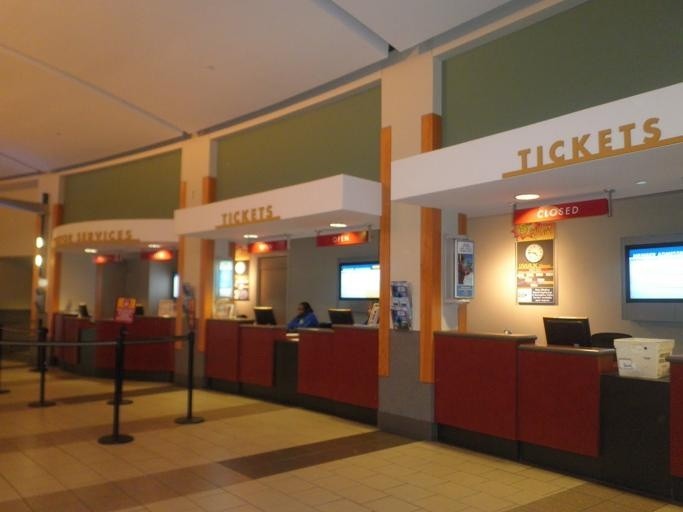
[78,304,88,318]
[543,317,592,347]
[338,261,380,301]
[619,232,683,323]
[253,307,277,325]
[171,271,180,299]
[327,309,355,325]
[134,304,143,315]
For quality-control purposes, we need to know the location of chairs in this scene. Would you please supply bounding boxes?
[592,333,632,349]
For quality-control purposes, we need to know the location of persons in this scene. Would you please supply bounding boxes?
[287,301,321,328]
[458,255,471,284]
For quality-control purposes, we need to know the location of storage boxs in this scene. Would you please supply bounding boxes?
[614,337,674,379]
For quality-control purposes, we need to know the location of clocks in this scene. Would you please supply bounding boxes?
[525,244,544,263]
